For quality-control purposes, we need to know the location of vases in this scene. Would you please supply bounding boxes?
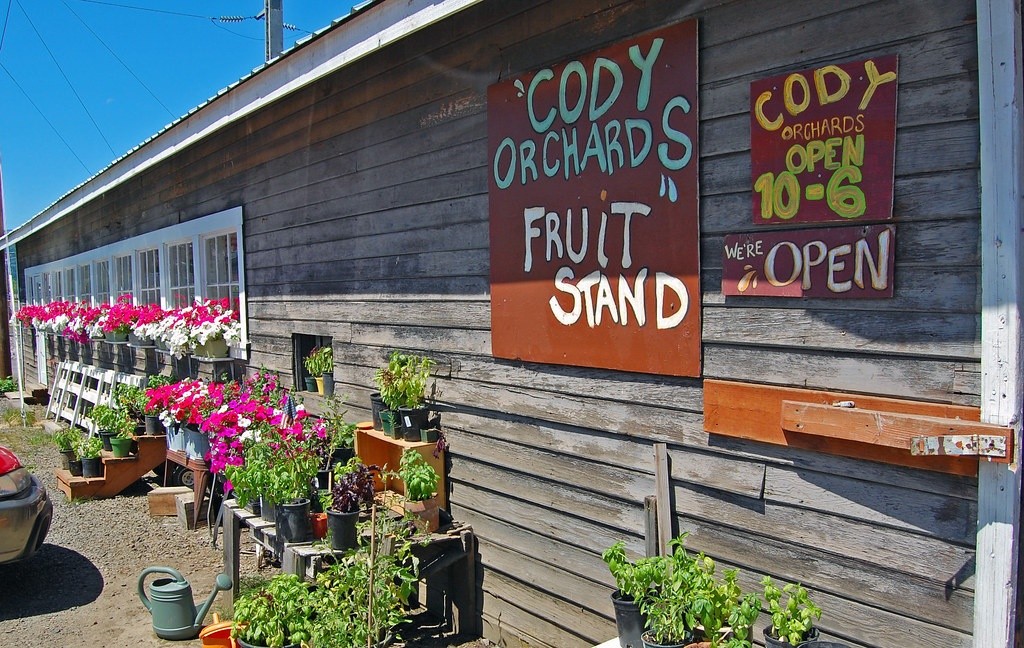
[232,492,260,514]
[105,333,127,342]
[165,426,185,453]
[129,334,153,346]
[260,487,276,522]
[194,340,229,358]
[157,337,167,350]
[276,499,310,543]
[183,423,210,460]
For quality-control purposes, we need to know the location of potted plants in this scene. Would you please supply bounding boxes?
[231,347,452,648]
[55,374,174,477]
[602,531,821,648]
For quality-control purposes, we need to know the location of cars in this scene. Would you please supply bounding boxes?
[0,446,54,567]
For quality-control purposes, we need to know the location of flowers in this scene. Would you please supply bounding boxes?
[144,363,328,509]
[16,294,241,360]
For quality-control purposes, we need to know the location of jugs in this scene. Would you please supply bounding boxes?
[137,566,232,641]
